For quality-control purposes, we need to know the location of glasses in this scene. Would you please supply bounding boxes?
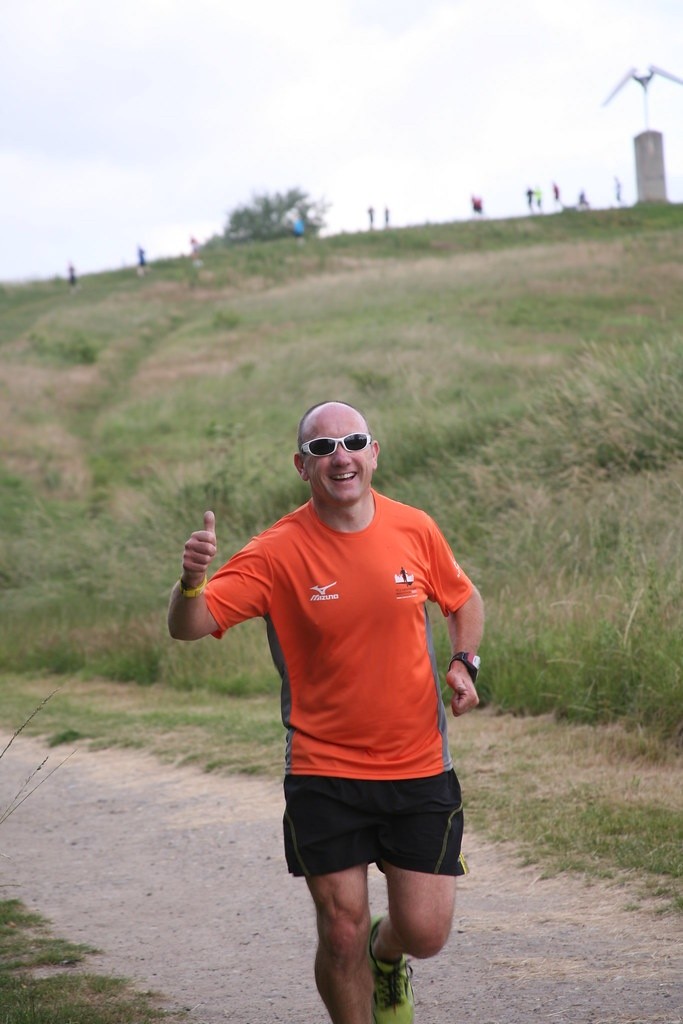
[300,431,373,458]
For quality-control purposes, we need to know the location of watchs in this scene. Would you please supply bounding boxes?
[447,652,481,683]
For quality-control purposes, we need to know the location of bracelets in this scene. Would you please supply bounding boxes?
[178,573,207,597]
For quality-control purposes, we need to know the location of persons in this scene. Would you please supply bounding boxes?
[552,182,563,213]
[191,238,203,267]
[580,194,590,210]
[290,214,305,245]
[168,400,485,1024]
[526,185,543,216]
[137,244,150,275]
[367,206,389,229]
[471,194,487,220]
[66,263,82,287]
[614,178,621,202]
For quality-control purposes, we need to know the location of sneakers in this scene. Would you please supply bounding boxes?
[367,915,416,1024]
